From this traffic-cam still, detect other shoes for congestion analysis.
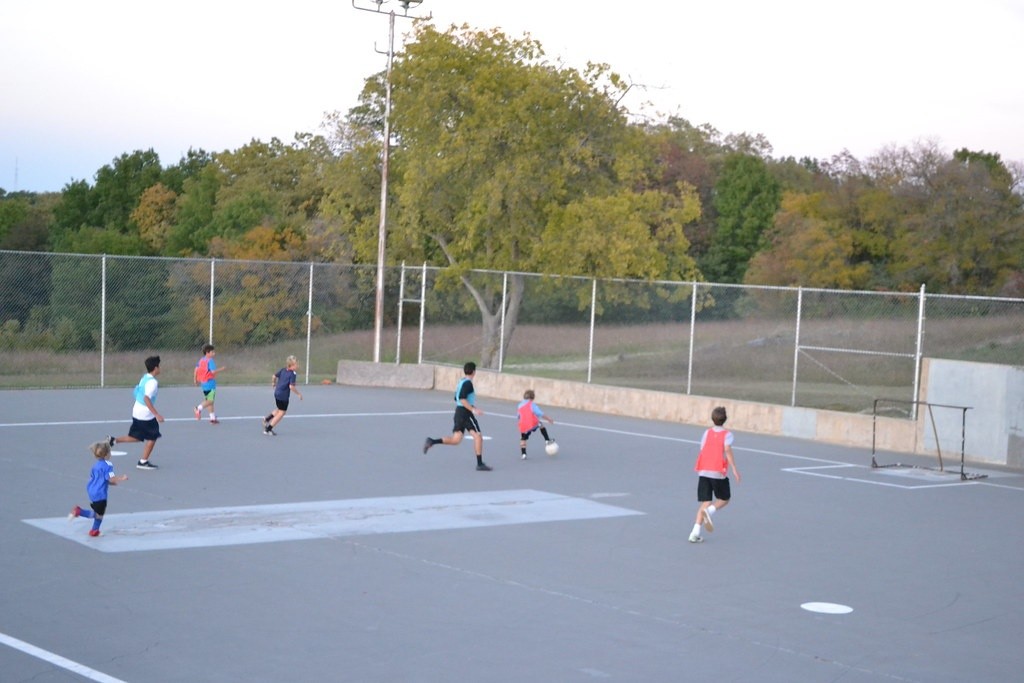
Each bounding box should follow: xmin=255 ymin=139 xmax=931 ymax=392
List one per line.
xmin=546 ymin=438 xmax=555 ymax=445
xmin=68 ymin=506 xmax=80 ymax=521
xmin=521 ymin=454 xmax=526 ymax=459
xmin=263 ymin=430 xmax=277 ymax=436
xmin=263 ymin=416 xmax=268 ymax=428
xmin=89 ymin=530 xmax=104 ymax=537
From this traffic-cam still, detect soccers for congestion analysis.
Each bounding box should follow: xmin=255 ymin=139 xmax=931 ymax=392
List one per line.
xmin=545 ymin=442 xmax=559 ymax=456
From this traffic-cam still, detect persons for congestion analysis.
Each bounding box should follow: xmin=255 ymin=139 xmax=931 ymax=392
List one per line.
xmin=688 ymin=407 xmax=740 ymax=544
xmin=516 ymin=390 xmax=555 ymax=460
xmin=67 ymin=440 xmax=129 ymax=537
xmin=192 ymin=343 xmax=227 ymax=424
xmin=423 ymin=361 xmax=493 ymax=471
xmin=107 ymin=356 xmax=164 ymax=469
xmin=262 ymin=355 xmax=302 ymax=436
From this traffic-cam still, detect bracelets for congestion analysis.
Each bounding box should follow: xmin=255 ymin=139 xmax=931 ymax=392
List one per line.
xmin=472 ymin=408 xmax=476 ymax=413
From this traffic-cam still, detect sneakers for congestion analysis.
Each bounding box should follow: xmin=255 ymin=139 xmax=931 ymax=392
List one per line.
xmin=210 ymin=420 xmax=219 ymax=424
xmin=476 ymin=463 xmax=492 ymax=470
xmin=689 ymin=534 xmax=703 ymax=542
xmin=136 ymin=461 xmax=158 ymax=470
xmin=423 ymin=438 xmax=432 ymax=454
xmin=701 ymin=509 xmax=713 ymax=532
xmin=106 ymin=435 xmax=115 ymax=448
xmin=194 ymin=406 xmax=201 ymax=420
